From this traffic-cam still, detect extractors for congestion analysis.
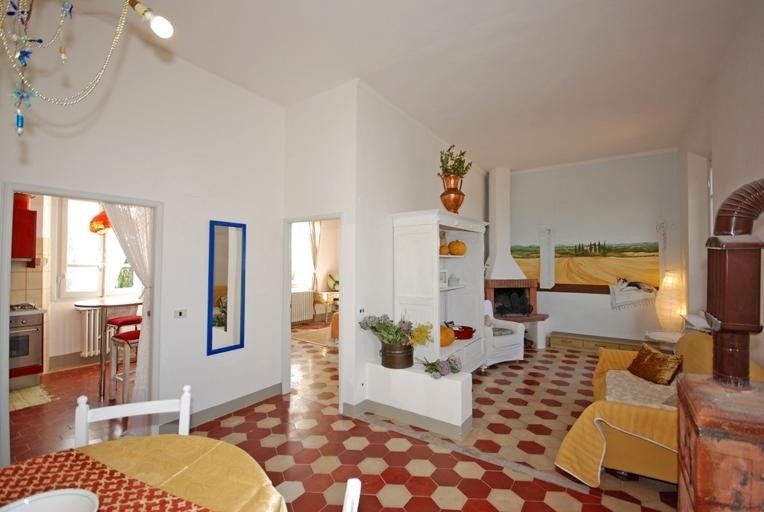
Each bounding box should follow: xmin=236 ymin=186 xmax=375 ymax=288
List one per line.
xmin=11 ymin=193 xmax=32 ymax=262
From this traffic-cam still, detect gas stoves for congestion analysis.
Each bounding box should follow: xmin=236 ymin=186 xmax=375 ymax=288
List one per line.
xmin=8 ymin=303 xmax=42 ymax=328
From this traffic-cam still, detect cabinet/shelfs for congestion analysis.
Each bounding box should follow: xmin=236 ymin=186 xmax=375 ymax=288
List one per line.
xmin=549 ymin=331 xmax=656 ymax=352
xmin=390 ymin=209 xmax=489 ymax=379
xmin=11 ymin=209 xmax=36 ymax=268
xmin=313 ymin=291 xmax=340 ymax=325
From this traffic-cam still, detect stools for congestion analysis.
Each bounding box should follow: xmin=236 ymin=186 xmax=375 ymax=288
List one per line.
xmin=110 ymin=330 xmax=141 ymax=403
xmin=104 ymin=315 xmax=142 ymax=362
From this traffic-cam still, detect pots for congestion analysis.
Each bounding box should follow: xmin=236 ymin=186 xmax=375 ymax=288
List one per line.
xmin=444 ymin=320 xmax=476 ymax=339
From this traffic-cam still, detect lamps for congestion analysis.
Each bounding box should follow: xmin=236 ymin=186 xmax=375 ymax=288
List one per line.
xmin=654 ymin=271 xmax=685 ymax=332
xmin=0 ymin=0 xmax=174 ymax=136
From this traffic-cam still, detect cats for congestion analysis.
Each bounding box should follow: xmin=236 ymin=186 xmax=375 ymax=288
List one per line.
xmin=616 ymin=277 xmax=656 ymax=293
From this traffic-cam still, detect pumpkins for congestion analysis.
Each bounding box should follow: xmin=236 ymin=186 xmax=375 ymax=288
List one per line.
xmin=440 ymin=325 xmax=454 ymax=346
xmin=448 ymin=240 xmax=466 ymax=255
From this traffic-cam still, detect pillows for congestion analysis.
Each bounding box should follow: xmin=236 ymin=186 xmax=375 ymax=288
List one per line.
xmin=627 ymin=342 xmax=682 ymax=385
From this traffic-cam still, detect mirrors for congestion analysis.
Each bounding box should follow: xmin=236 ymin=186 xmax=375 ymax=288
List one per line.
xmin=205 ymin=218 xmax=248 ymax=356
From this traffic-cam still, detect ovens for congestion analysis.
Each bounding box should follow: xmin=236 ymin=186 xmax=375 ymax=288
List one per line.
xmin=8 ymin=326 xmax=42 ymax=390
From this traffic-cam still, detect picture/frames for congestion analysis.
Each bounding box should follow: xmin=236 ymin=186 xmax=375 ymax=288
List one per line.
xmin=440 ymin=269 xmax=448 ymax=289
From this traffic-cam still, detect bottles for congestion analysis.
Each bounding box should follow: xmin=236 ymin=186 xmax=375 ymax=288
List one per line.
xmin=447 ymin=275 xmax=457 ymax=286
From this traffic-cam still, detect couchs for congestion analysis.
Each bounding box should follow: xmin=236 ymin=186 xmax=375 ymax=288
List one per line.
xmin=553 ymin=331 xmax=763 ymax=489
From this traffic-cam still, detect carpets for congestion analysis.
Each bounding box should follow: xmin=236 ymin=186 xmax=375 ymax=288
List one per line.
xmin=9 ymin=384 xmax=62 ymax=413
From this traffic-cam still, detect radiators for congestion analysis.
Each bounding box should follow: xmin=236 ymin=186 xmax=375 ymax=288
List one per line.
xmin=75 ymin=306 xmax=122 ymax=358
xmin=291 ymin=290 xmax=314 ymax=325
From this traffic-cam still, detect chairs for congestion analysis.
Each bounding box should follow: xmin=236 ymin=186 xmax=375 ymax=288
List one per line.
xmin=71 ymin=384 xmax=190 ymax=448
xmin=324 ymin=312 xmax=339 ymax=358
xmin=483 ymin=300 xmax=525 ymax=366
xmin=339 ymin=478 xmax=365 ymax=511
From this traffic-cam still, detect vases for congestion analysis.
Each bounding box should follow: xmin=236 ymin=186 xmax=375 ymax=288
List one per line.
xmin=436 ymin=172 xmax=465 ymax=214
xmin=377 ymin=341 xmax=415 ymax=370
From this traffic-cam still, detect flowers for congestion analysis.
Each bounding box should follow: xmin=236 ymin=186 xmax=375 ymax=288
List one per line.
xmin=416 ymin=352 xmax=464 ymax=379
xmin=437 ymin=144 xmax=473 ymax=176
xmin=216 ymin=295 xmax=227 ymax=312
xmin=211 ymin=313 xmax=225 ymax=327
xmin=358 ymin=309 xmax=435 ymax=349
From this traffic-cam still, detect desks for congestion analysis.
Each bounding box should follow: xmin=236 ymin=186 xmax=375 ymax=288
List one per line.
xmin=76 ymin=296 xmax=143 ymax=397
